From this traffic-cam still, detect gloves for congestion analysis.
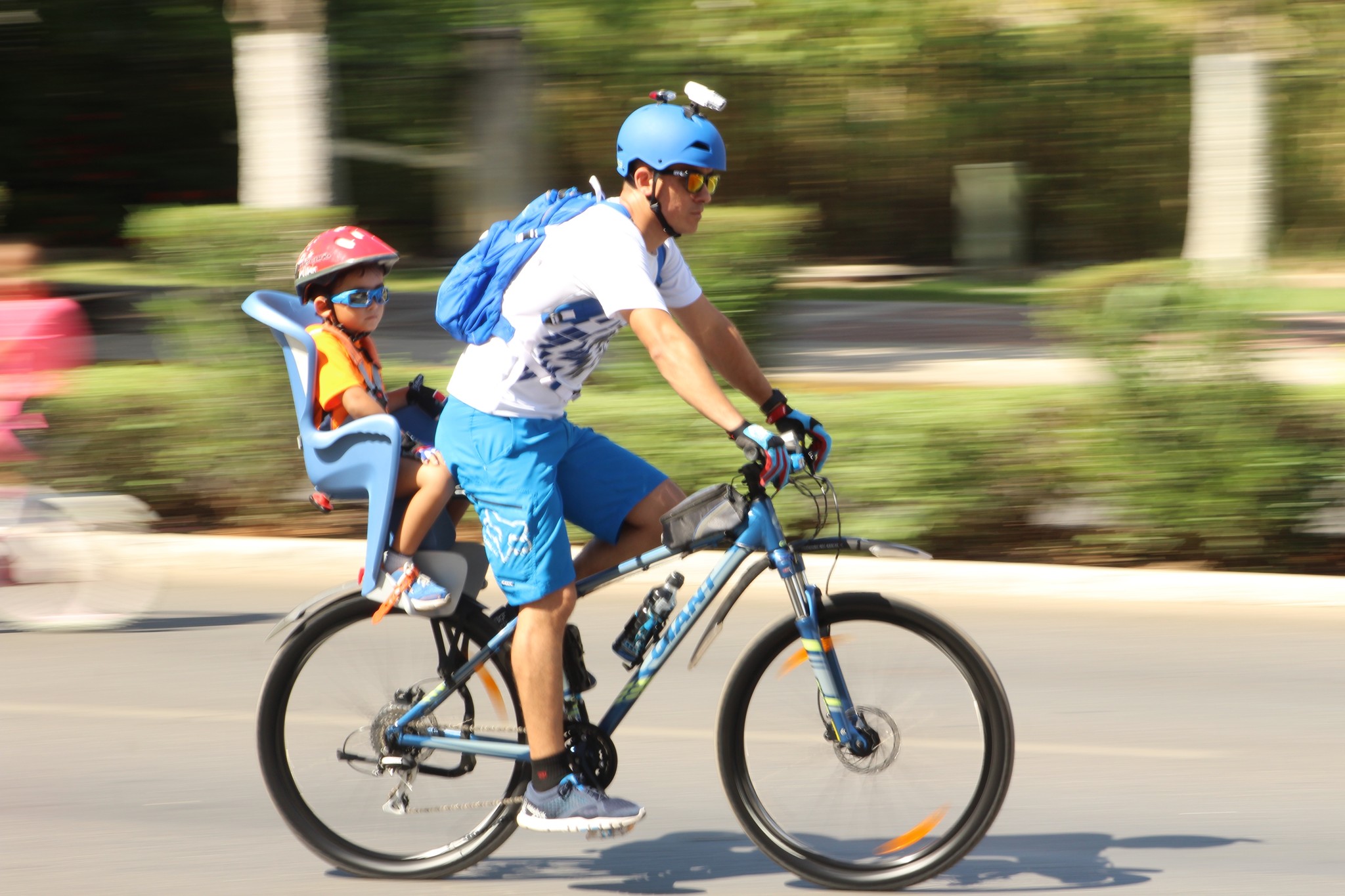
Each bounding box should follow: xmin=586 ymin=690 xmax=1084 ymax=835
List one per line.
xmin=768 ymin=404 xmax=833 ymax=475
xmin=727 ymin=420 xmax=792 ymax=498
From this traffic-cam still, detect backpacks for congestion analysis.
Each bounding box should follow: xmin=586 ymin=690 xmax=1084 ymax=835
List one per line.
xmin=435 ymin=175 xmax=666 ymax=404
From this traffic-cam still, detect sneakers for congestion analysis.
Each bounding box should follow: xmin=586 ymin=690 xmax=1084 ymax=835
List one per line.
xmin=515 ymin=769 xmax=646 ymax=832
xmin=380 ymin=561 xmax=452 ymax=611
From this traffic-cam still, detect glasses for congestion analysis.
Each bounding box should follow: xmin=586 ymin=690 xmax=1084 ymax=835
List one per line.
xmin=649 ymin=167 xmax=721 ymax=195
xmin=330 ymin=287 xmax=390 ymax=308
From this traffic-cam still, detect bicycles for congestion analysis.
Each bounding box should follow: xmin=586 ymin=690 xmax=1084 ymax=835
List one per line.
xmin=257 ymin=426 xmax=1017 ymax=893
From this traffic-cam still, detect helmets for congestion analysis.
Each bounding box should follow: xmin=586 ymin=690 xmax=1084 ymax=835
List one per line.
xmin=293 ymin=225 xmax=403 ymax=306
xmin=615 ymin=102 xmax=727 ymax=178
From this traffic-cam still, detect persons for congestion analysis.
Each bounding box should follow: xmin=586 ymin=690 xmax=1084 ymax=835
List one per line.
xmin=0 ymin=242 xmax=97 ymax=462
xmin=436 ymin=103 xmax=832 ymax=834
xmin=294 ymin=226 xmax=470 ymax=613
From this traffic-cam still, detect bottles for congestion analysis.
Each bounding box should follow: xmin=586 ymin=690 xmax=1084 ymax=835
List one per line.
xmin=610 ymin=571 xmax=685 ymax=669
